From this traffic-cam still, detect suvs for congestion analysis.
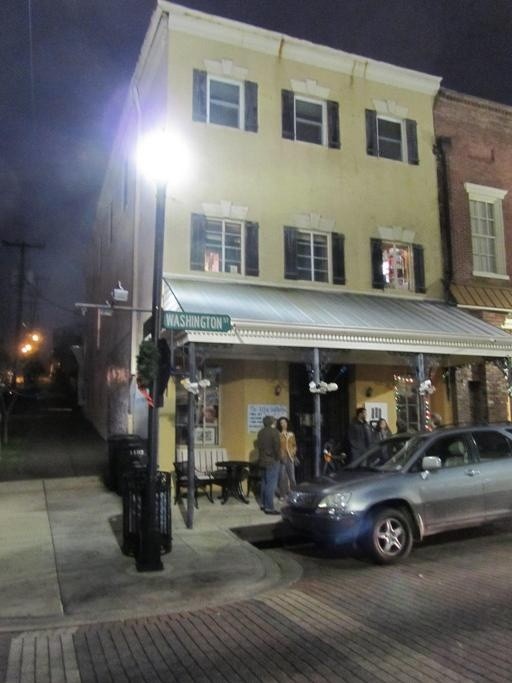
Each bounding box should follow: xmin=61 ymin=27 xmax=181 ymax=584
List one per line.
xmin=280 ymin=421 xmax=511 ymax=563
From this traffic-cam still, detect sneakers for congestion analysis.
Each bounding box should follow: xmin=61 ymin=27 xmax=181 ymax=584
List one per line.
xmin=261 ymin=507 xmax=280 ymax=515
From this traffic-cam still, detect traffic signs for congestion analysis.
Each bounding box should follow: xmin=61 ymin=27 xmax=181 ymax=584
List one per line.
xmin=162 ymin=312 xmax=232 ymax=332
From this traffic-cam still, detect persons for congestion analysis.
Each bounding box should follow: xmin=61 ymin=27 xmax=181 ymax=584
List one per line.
xmin=372 ymin=419 xmax=393 ymax=465
xmin=347 ymin=408 xmax=373 ymax=467
xmin=275 ymin=417 xmax=298 ymax=502
xmin=396 ymin=418 xmax=412 ymax=452
xmin=257 ymin=416 xmax=285 ymax=514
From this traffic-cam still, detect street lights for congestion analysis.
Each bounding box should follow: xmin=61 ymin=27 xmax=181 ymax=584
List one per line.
xmin=129 ymin=119 xmax=200 ymax=574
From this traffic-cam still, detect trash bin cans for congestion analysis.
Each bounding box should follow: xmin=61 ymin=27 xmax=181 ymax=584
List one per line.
xmin=107 ymin=434 xmax=172 ymax=558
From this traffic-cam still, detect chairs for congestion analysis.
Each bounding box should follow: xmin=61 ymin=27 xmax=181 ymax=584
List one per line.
xmin=173 ymin=459 xmax=261 ymax=510
